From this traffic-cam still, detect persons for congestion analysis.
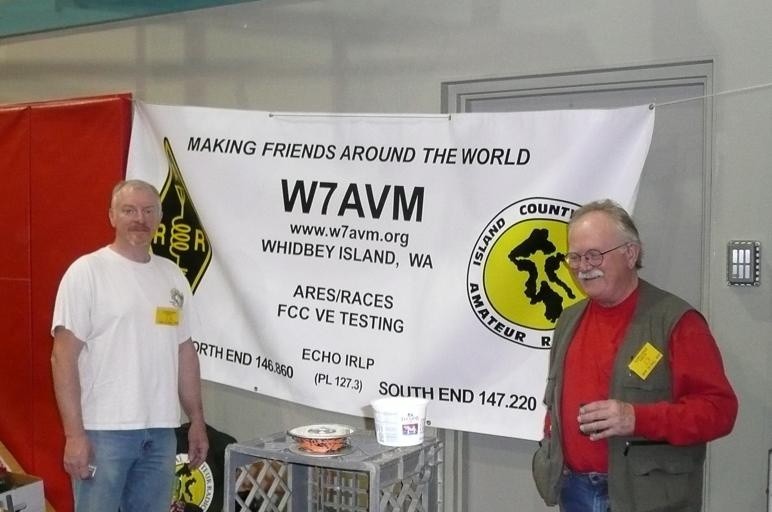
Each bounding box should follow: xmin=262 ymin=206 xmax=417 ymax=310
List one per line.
xmin=528 ymin=197 xmax=740 ymax=512
xmin=47 ymin=177 xmax=210 ymax=509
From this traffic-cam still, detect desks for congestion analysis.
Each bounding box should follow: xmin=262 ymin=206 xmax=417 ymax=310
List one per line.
xmin=220 ymin=422 xmax=444 ymax=511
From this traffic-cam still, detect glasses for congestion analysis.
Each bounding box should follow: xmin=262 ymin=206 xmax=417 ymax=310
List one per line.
xmin=563 ymin=243 xmax=630 ymax=269
